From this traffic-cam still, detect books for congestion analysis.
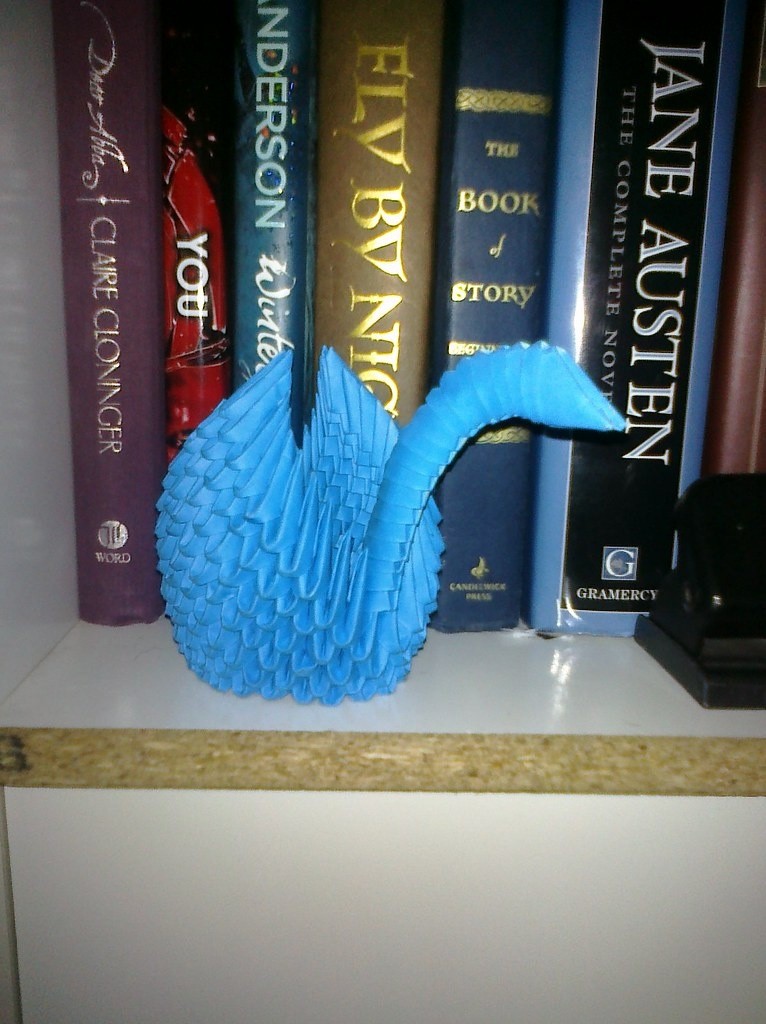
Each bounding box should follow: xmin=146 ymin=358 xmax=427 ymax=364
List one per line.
xmin=50 ymin=0 xmax=766 ymax=638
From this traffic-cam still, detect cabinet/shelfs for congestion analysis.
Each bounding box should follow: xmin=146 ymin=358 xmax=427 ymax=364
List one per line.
xmin=0 ymin=0 xmax=766 ymax=1023
